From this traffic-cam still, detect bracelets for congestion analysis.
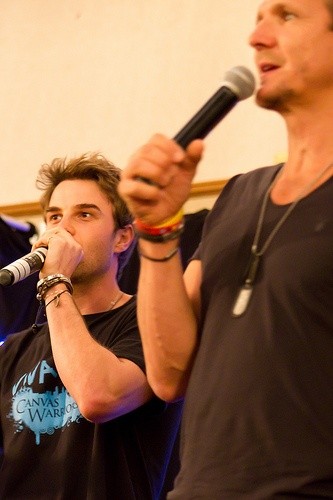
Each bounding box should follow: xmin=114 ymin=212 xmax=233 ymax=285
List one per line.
xmin=32 ymin=273 xmax=73 ymax=327
xmin=134 ymin=245 xmax=186 ymax=265
xmin=130 ymin=218 xmax=188 ymax=244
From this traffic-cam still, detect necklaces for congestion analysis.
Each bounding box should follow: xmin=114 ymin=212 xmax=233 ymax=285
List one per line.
xmin=230 ymin=162 xmax=333 ymax=316
xmin=99 ymin=291 xmax=124 ymax=317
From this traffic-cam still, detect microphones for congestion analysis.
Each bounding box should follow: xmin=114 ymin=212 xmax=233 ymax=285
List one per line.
xmin=0 ymin=246 xmax=47 ymax=287
xmin=133 ymin=66 xmax=256 ymax=188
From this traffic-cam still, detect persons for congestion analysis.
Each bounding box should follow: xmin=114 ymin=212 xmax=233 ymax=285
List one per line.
xmin=1 ymin=153 xmax=185 ymax=500
xmin=118 ymin=0 xmax=333 ymax=500
